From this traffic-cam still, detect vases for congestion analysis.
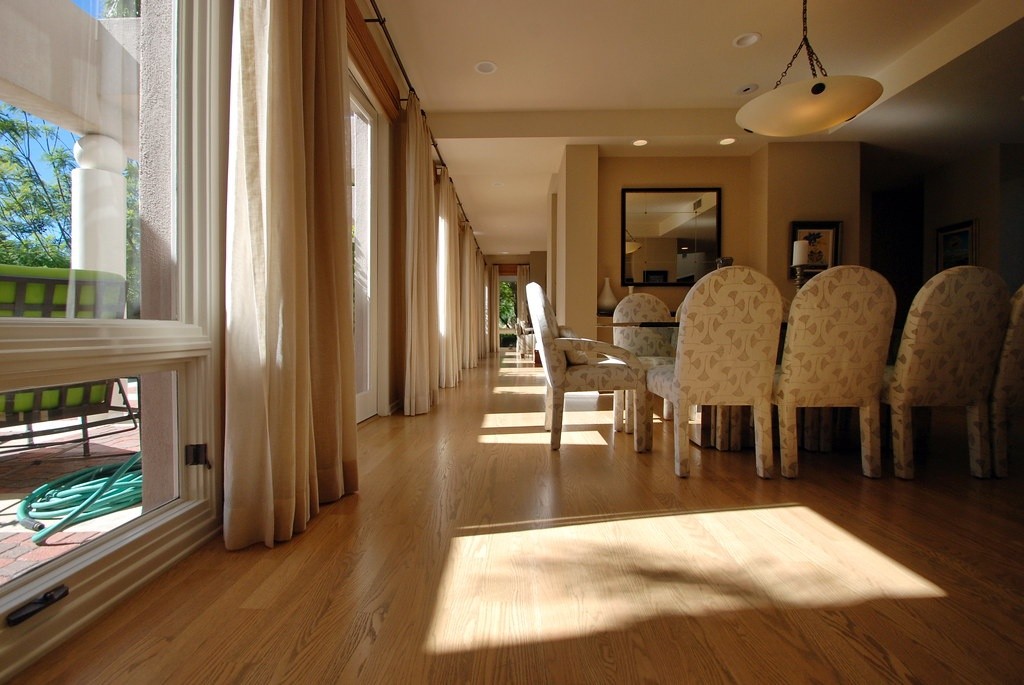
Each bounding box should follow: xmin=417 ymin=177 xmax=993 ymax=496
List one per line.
xmin=598 ymin=277 xmax=619 ymax=314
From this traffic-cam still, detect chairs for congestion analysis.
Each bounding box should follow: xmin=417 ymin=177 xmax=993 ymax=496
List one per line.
xmin=524 ymin=281 xmax=650 ymax=453
xmin=646 ymin=266 xmax=783 ymax=480
xmin=613 ymin=293 xmax=678 ymax=433
xmin=993 ymin=285 xmax=1024 ymax=478
xmin=771 ymin=263 xmax=896 ymax=480
xmin=882 ymin=265 xmax=1012 ymax=483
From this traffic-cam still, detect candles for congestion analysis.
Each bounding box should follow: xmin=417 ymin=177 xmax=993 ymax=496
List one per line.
xmin=790 ymin=239 xmax=810 ymax=293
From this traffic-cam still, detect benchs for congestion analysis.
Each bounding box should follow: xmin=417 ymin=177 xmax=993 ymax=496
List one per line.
xmin=0 ymin=264 xmax=141 ymax=463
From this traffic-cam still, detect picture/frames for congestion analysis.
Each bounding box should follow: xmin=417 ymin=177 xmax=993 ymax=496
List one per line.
xmin=787 ymin=219 xmax=844 ymax=281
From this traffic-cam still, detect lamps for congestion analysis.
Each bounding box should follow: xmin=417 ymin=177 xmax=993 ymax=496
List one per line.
xmin=734 ymin=1 xmax=884 ymax=138
xmin=625 ymin=229 xmax=641 ymax=255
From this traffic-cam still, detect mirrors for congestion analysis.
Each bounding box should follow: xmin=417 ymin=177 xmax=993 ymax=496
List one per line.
xmin=621 ymin=186 xmax=724 ymax=288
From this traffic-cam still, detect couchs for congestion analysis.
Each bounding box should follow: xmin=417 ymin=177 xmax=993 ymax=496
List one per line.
xmin=515 ymin=317 xmax=536 ymax=359
